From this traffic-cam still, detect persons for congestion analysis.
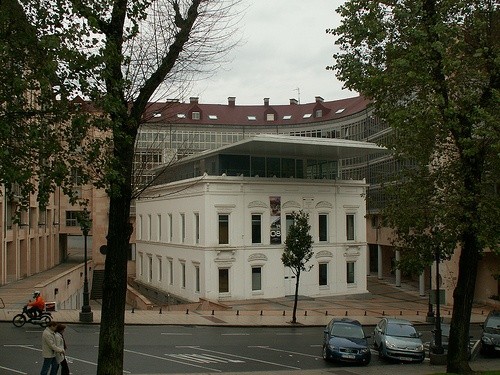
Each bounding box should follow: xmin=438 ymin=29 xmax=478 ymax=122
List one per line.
xmin=54 ymin=325 xmax=70 ymax=375
xmin=27 ymin=291 xmax=45 ymax=318
xmin=40 ymin=321 xmax=64 ymax=375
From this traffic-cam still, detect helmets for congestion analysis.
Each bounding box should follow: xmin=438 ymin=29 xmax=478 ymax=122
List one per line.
xmin=33 ymin=291 xmax=40 ymax=297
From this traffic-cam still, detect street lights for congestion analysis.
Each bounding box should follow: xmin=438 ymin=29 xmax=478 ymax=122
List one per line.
xmin=79 ymin=218 xmax=94 ymax=323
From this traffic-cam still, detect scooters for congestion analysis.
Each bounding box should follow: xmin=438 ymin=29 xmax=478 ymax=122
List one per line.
xmin=12 ymin=300 xmax=54 ymax=328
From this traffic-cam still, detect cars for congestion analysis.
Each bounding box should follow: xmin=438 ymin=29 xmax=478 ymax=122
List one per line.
xmin=323 ymin=317 xmax=371 ymax=367
xmin=479 ymin=309 xmax=500 ymax=361
xmin=373 ymin=318 xmax=426 ymax=366
